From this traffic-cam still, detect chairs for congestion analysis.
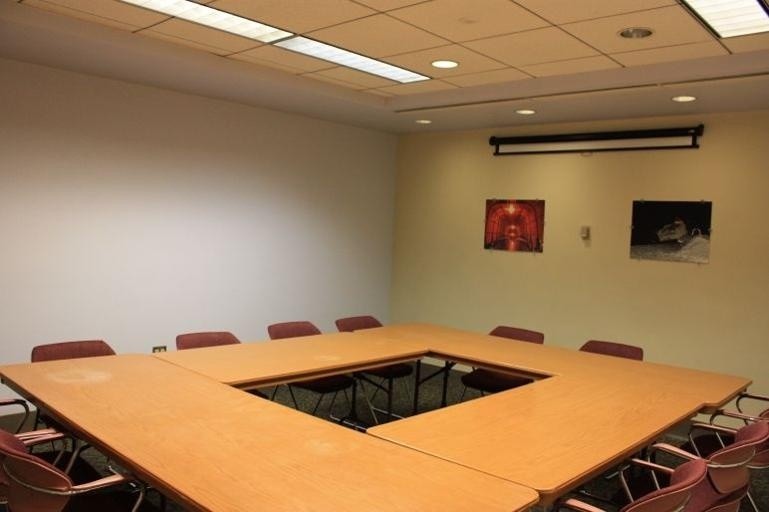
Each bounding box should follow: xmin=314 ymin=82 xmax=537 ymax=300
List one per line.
xmin=333 ymin=315 xmax=416 ymax=420
xmin=30 ymin=340 xmax=117 ymax=465
xmin=268 ymin=321 xmax=378 ymax=431
xmin=681 ymin=390 xmax=768 ymax=470
xmin=579 ymin=339 xmax=645 ymax=360
xmin=1 ymin=399 xmax=91 ymax=465
xmin=554 ymin=455 xmax=708 ymax=512
xmin=490 ymin=325 xmax=544 ymax=345
xmin=178 ymin=332 xmax=269 ymax=399
xmin=639 ymin=421 xmax=768 ymax=511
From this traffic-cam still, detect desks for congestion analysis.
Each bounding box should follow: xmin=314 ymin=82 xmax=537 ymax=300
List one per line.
xmin=366 ymin=375 xmax=706 ymax=510
xmin=150 ymin=331 xmax=429 ymax=390
xmin=353 ymin=321 xmax=754 ymax=415
xmin=1 ymin=352 xmax=541 ymax=511
